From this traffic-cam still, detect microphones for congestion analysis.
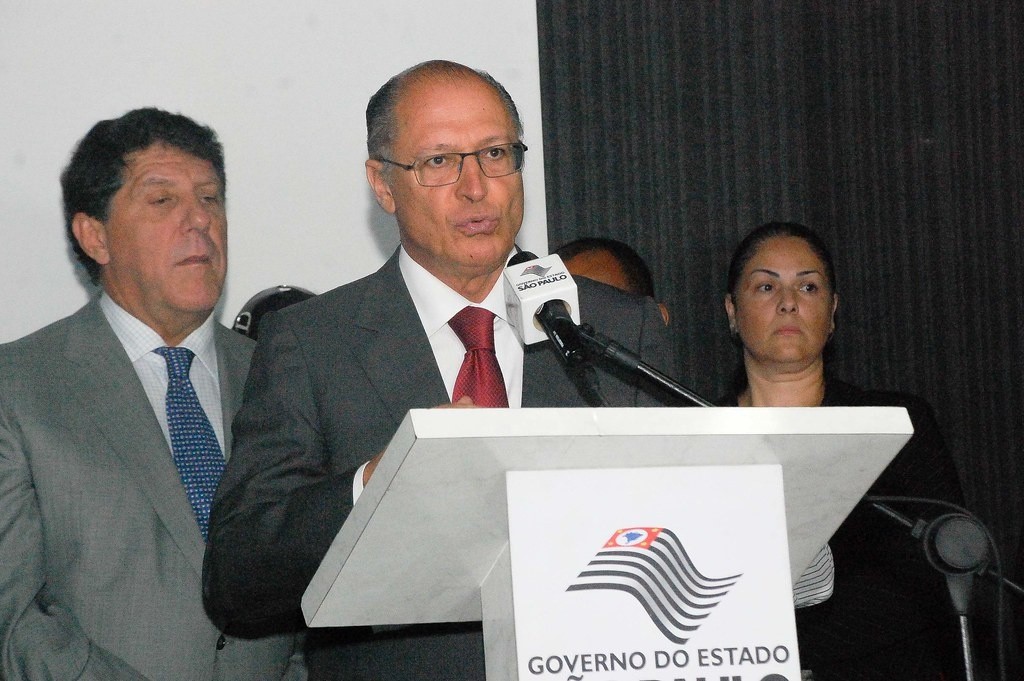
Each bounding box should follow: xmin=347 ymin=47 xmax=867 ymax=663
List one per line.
xmin=500 ymin=251 xmax=587 ymax=368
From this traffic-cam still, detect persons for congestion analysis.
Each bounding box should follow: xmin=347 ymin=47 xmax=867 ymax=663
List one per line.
xmin=0 ymin=108 xmax=310 ymax=681
xmin=710 ymin=220 xmax=997 ymax=681
xmin=200 ymin=60 xmax=681 ymax=681
xmin=551 ymin=236 xmax=669 ymax=327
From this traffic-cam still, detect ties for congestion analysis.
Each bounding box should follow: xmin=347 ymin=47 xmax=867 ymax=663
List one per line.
xmin=447 ymin=307 xmax=509 ymax=409
xmin=151 ymin=347 xmax=228 ymax=542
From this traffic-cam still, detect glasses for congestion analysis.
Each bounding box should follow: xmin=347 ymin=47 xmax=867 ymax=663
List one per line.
xmin=379 ymin=140 xmax=528 ymax=187
